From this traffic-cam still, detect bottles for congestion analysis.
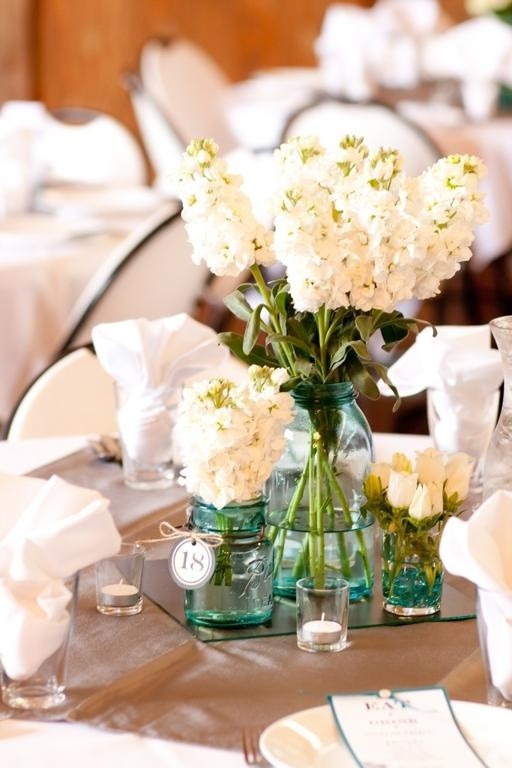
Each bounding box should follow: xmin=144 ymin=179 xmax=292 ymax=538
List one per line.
xmin=484 ymin=315 xmax=512 ymax=500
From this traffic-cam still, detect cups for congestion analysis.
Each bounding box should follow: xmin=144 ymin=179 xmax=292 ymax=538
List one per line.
xmin=114 ymin=378 xmax=179 ymax=489
xmin=296 ymin=577 xmax=349 ymax=654
xmin=1 ymin=568 xmax=78 ymax=709
xmin=94 ymin=543 xmax=146 ymax=616
xmin=427 ymin=389 xmax=500 ymax=493
xmin=475 ymin=585 xmax=512 ymax=708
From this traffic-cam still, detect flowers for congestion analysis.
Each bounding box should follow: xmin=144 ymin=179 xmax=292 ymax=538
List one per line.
xmin=173 ymin=135 xmax=489 ymax=593
xmin=362 ymin=442 xmax=476 ymax=600
xmin=172 ymin=365 xmax=296 ymax=585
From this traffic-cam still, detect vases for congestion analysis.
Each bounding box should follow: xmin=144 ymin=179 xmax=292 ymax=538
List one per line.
xmin=183 ymin=499 xmax=275 ymax=628
xmin=260 ymin=381 xmax=375 ymax=600
xmin=377 ymin=529 xmax=443 ymax=619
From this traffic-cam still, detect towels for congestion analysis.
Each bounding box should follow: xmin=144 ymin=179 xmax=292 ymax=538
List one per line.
xmin=439 ymin=491 xmax=512 ymax=706
xmin=376 ymin=324 xmax=505 ymax=471
xmin=91 ymin=311 xmax=251 ymax=466
xmin=0 ymin=473 xmax=121 ymax=684
